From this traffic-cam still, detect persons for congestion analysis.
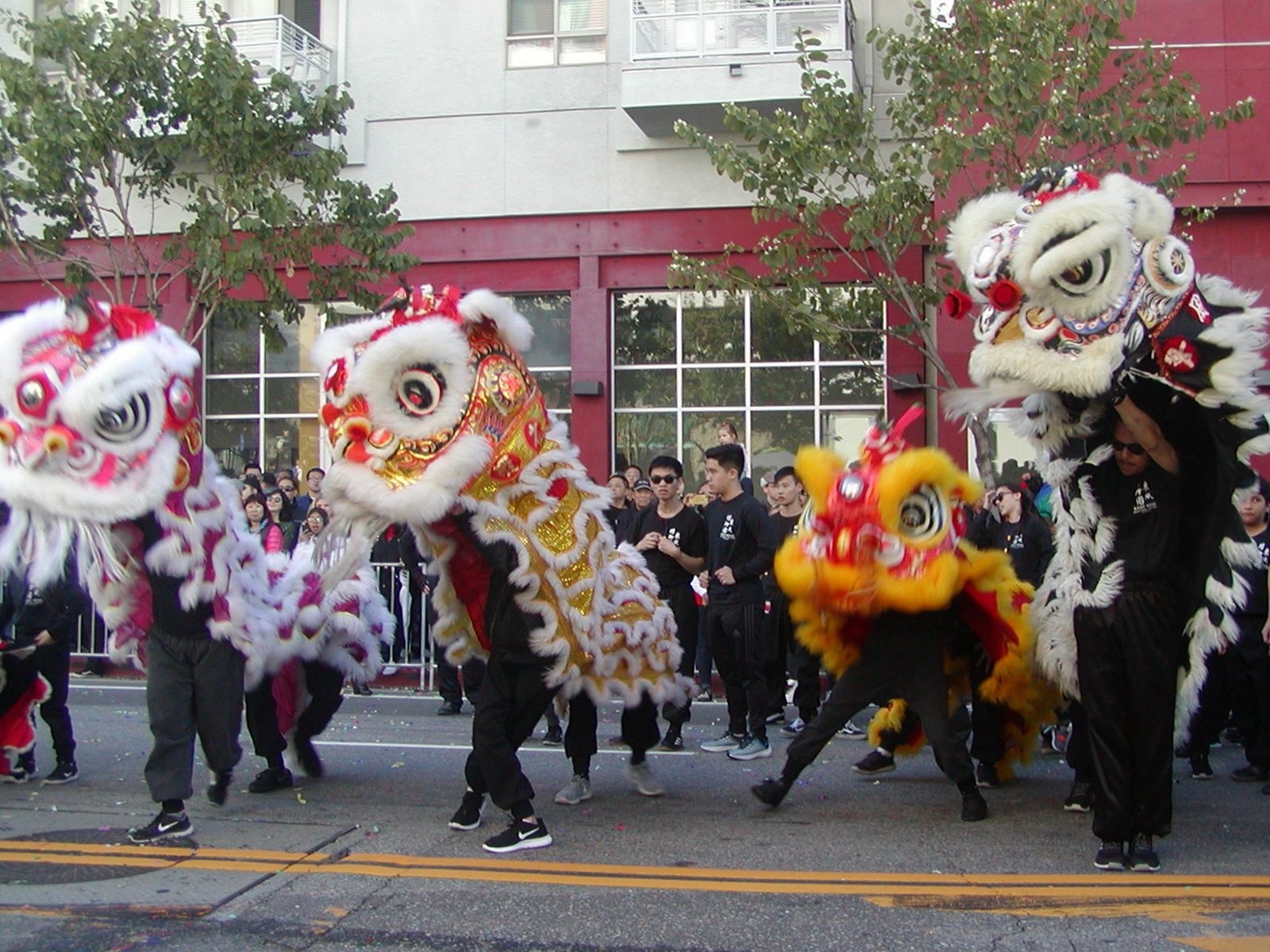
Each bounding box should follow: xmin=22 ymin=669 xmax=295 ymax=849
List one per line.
xmin=602 ymin=464 xmax=652 ymax=548
xmin=245 ymin=658 xmax=345 ymax=794
xmin=700 ymin=445 xmax=775 ymax=760
xmin=555 ymin=689 xmax=664 ymax=804
xmin=760 ymin=468 xmax=869 ymax=739
xmin=237 ymin=464 xmax=328 ymax=559
xmin=678 ymin=474 xmax=753 ymax=703
xmin=0 ymin=502 xmax=110 ymax=784
xmin=1063 ymin=700 xmax=1098 ymax=813
xmin=752 ymin=595 xmax=1010 ymax=821
xmin=1065 ymin=382 xmax=1193 ymax=871
xmin=448 ymin=510 xmax=567 ymax=854
xmin=1177 ymin=476 xmax=1270 ymax=795
xmin=128 ymin=512 xmax=249 ymax=842
xmin=969 ymin=469 xmax=1074 ymax=752
xmin=352 ymin=524 xmax=486 ymax=715
xmin=610 ymin=455 xmax=709 ymax=750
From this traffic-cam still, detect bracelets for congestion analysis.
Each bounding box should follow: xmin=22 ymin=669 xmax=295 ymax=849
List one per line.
xmin=1111 ymin=392 xmax=1126 ymax=405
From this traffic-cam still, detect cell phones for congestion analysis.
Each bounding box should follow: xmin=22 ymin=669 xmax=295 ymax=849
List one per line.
xmin=302 ymin=521 xmax=309 ymax=534
xmin=688 ymin=494 xmax=708 ymax=504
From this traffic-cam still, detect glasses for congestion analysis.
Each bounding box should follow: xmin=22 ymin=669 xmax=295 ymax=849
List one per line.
xmin=651 ymin=474 xmax=678 ymax=485
xmin=306 ymin=518 xmax=325 ymax=523
xmin=993 ymin=492 xmax=1014 ymax=506
xmin=266 ymin=497 xmax=283 ymax=502
xmin=279 ymin=486 xmax=295 ymax=491
xmin=1110 ymin=435 xmax=1143 ymax=455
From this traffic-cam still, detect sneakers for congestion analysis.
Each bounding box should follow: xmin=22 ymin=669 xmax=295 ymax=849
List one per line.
xmin=851 ymin=750 xmax=896 ymax=775
xmin=1 ymin=736 xmax=325 ymax=842
xmin=447 ymin=790 xmax=484 ymax=830
xmin=834 ymin=720 xmax=868 ymax=740
xmin=1063 ymin=776 xmax=1091 ymax=812
xmin=544 ymin=685 xmax=823 ymax=806
xmin=975 ymin=759 xmax=997 ymax=787
xmin=481 ymin=815 xmax=552 ymax=852
xmin=1093 ymin=836 xmax=1125 ymax=869
xmin=1126 ymin=831 xmax=1160 ymax=871
xmin=1189 ymin=749 xmax=1213 ymax=779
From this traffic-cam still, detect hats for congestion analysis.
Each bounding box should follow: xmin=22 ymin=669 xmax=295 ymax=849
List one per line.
xmin=634 ymin=479 xmax=652 ymax=491
xmin=760 ymin=472 xmax=779 ymax=488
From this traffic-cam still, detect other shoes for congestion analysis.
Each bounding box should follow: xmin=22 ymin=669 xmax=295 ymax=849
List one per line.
xmin=1263 ymin=780 xmax=1270 ymax=796
xmin=1053 ymin=725 xmax=1068 ymax=753
xmin=1231 ymin=765 xmax=1264 ymax=781
xmin=1040 ymin=735 xmax=1055 ymax=754
xmin=349 ymin=645 xmax=464 ymax=715
xmin=961 ymin=788 xmax=985 ymax=822
xmin=67 ymin=670 xmax=102 ymax=679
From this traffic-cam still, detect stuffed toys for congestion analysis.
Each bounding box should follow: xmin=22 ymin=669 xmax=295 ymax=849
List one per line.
xmin=775 ymin=402 xmax=1056 ymax=783
xmin=309 ymin=284 xmax=700 ymax=708
xmin=0 ymin=292 xmax=397 ymax=745
xmin=937 ymin=174 xmax=1270 ymax=742
